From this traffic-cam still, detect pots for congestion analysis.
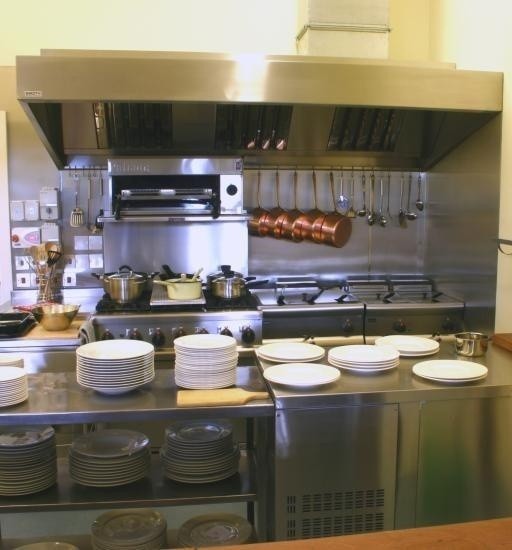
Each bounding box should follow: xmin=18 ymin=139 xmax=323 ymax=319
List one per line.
xmin=92 ymin=265 xmax=269 ymax=303
xmin=249 ymin=169 xmax=352 ymax=248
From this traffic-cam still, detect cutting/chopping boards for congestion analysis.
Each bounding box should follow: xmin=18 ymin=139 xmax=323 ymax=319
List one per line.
xmin=176 ymin=388 xmax=269 ymax=407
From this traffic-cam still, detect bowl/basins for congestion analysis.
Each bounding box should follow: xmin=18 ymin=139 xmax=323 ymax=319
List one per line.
xmin=32 ymin=304 xmax=81 ymax=332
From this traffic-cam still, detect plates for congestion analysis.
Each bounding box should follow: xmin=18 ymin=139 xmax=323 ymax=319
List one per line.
xmin=76 ymin=338 xmax=157 ymax=396
xmin=173 ymin=333 xmax=238 ymax=390
xmin=0 ymin=418 xmax=242 ymax=494
xmin=256 ymin=332 xmax=489 ymax=387
xmin=10 ymin=507 xmax=256 ymax=550
xmin=1 ymin=352 xmax=30 ymax=409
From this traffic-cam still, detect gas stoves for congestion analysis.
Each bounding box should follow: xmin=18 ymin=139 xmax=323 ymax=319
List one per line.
xmin=91 ymin=302 xmax=263 ymax=349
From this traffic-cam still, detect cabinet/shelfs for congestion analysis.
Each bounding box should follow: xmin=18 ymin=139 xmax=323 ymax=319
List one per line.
xmin=1 ymin=365 xmax=275 ymax=550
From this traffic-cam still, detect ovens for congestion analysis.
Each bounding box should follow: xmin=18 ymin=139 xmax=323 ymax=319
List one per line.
xmin=108 ymin=156 xmax=244 ymax=220
